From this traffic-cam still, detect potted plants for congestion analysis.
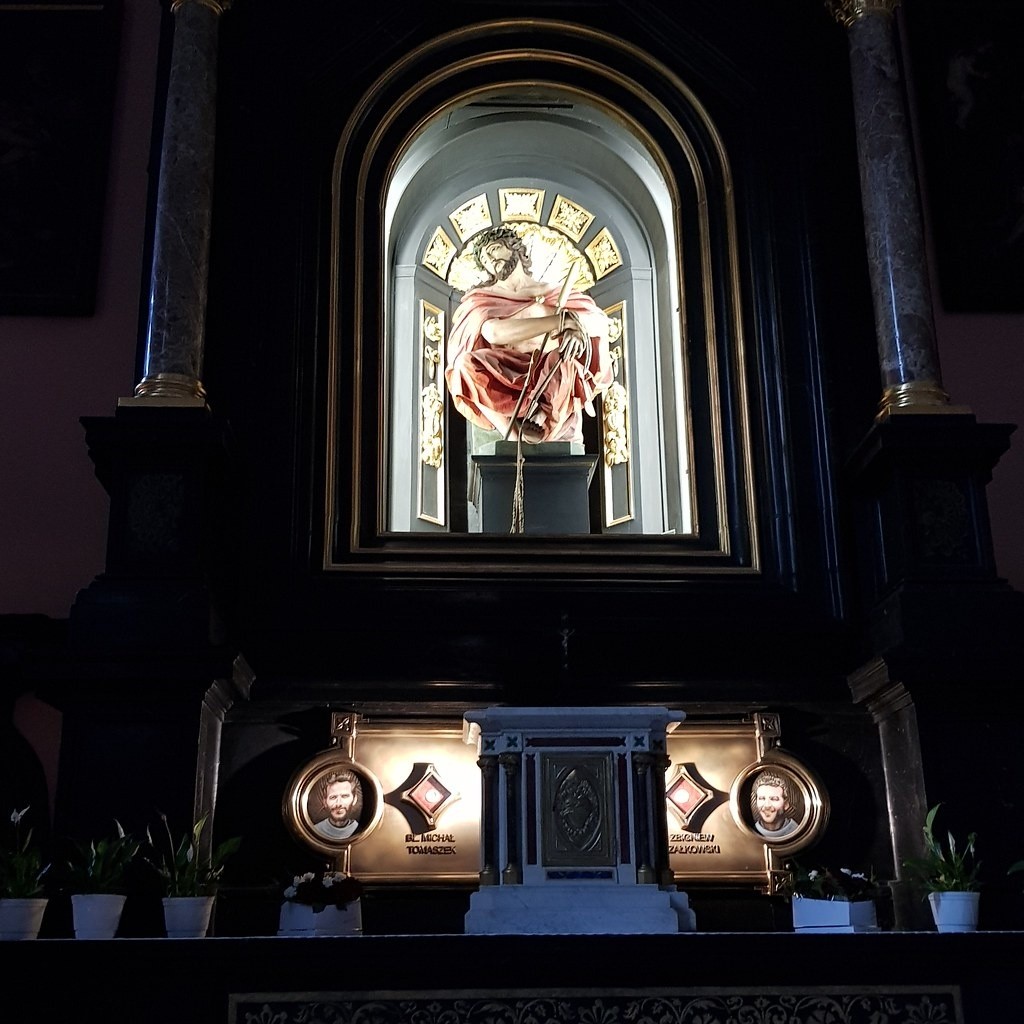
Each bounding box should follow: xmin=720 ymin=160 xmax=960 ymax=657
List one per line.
xmin=141 ymin=809 xmax=243 ymax=937
xmin=904 ymin=801 xmax=989 ymax=932
xmin=60 ymin=818 xmax=139 ymax=938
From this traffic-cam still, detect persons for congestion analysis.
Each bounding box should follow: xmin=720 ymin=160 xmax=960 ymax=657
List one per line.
xmin=437 ymin=229 xmax=613 ymax=443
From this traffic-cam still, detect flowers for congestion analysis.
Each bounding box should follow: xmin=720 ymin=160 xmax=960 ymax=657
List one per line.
xmin=0 ymin=805 xmax=52 ymax=899
xmin=784 ymin=859 xmax=877 ymax=901
xmin=285 ymin=870 xmax=363 ymax=914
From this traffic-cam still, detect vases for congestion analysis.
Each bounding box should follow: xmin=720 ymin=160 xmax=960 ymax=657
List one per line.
xmin=0 ymin=900 xmax=49 ymax=940
